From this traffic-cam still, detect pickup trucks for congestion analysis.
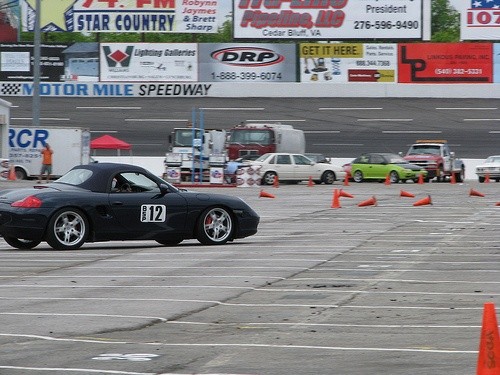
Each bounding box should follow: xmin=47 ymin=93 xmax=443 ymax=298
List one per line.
xmin=398 ymin=143 xmax=465 ymax=182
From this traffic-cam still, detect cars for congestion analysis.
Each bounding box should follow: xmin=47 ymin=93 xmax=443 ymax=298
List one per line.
xmin=343 ymin=151 xmax=427 ymax=183
xmin=254 ymin=153 xmax=346 ymax=185
xmin=475 ymin=155 xmax=500 ymax=183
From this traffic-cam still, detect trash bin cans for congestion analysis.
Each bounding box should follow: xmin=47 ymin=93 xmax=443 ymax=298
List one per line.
xmin=209 ymin=154 xmax=226 ymax=184
xmin=166 ymin=154 xmax=183 ymax=184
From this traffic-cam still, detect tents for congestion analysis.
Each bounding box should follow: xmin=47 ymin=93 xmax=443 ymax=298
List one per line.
xmin=90 ymin=135 xmax=132 ymax=155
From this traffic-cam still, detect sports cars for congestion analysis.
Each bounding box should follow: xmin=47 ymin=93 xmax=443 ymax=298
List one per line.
xmin=0 ymin=162 xmax=259 ymax=250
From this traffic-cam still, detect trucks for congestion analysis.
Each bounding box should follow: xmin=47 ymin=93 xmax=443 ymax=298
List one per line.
xmin=169 ymin=126 xmax=229 ymax=172
xmin=225 ymin=122 xmax=306 ymax=159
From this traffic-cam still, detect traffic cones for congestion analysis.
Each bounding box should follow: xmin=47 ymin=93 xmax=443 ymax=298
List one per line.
xmin=272 ymin=175 xmax=280 ymax=187
xmin=308 ymin=176 xmax=315 ymax=187
xmin=400 ymin=189 xmax=431 ymax=207
xmin=329 ymin=188 xmax=354 ymax=208
xmin=449 ymin=173 xmax=457 ymax=184
xmin=484 ymin=173 xmax=491 ymax=183
xmin=259 ymin=190 xmax=276 ymax=199
xmin=7 ymin=163 xmax=18 ymax=180
xmin=470 ymin=188 xmax=484 ymax=197
xmin=358 ymin=196 xmax=377 ymax=207
xmin=476 ymin=303 xmax=500 ymax=375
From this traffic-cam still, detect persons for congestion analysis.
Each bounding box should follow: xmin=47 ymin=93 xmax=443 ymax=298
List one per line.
xmin=38 ymin=143 xmax=53 ymax=184
